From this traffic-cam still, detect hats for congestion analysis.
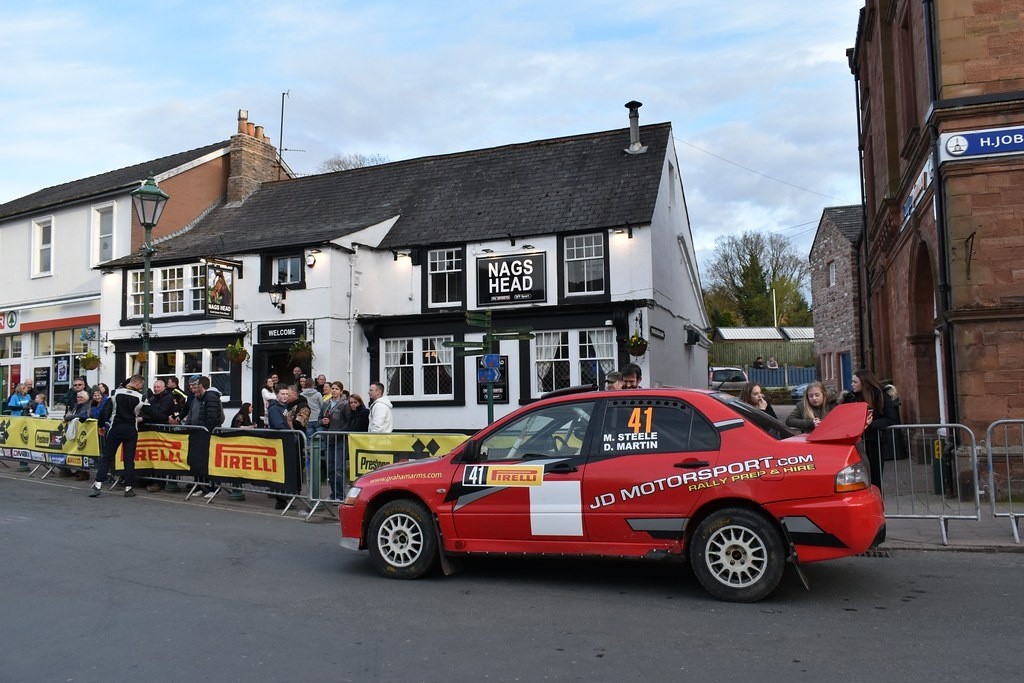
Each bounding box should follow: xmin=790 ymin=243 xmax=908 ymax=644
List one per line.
xmin=91 ymin=384 xmax=105 ymax=397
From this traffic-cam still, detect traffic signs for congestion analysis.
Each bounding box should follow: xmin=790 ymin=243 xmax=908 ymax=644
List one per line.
xmin=466 ymin=318 xmax=487 ymax=327
xmin=495 ymin=325 xmax=535 ymax=334
xmin=480 ymin=354 xmax=501 ymax=369
xmin=493 ymin=333 xmax=536 ymax=342
xmin=441 ymin=340 xmax=485 ymax=348
xmin=477 ymin=367 xmax=502 ymax=384
xmin=464 ymin=310 xmax=486 ymax=320
xmin=454 ymin=349 xmax=486 ymax=357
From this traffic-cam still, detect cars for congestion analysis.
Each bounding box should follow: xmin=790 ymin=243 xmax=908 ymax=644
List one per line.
xmin=336 ymin=384 xmax=891 ymax=606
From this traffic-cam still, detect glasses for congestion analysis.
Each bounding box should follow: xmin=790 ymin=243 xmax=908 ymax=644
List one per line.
xmin=73 ymin=383 xmax=83 ymax=386
xmin=298 ymin=373 xmax=307 ymax=377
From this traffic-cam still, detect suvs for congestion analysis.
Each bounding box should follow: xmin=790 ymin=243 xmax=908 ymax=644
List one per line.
xmin=708 ymin=366 xmax=749 ymax=396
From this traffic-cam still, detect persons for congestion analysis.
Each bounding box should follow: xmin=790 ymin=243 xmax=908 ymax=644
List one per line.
xmin=766 ymin=356 xmax=778 ymax=369
xmin=841 ymin=370 xmax=897 ymax=493
xmin=6 ymin=367 xmax=393 ymax=510
xmin=89 ymin=374 xmax=175 ymax=497
xmin=785 ymin=382 xmax=839 ymax=433
xmin=621 ymin=364 xmax=642 ymax=389
xmin=741 ymin=383 xmax=776 ymax=417
xmin=606 ymin=372 xmax=623 ymax=390
xmin=753 ymin=357 xmax=765 ymax=368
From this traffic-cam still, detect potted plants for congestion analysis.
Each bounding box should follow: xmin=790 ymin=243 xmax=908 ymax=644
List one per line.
xmin=288 ymin=335 xmax=316 ymax=370
xmin=80 ymin=349 xmax=101 ymax=370
xmin=224 ymin=338 xmax=250 ymax=369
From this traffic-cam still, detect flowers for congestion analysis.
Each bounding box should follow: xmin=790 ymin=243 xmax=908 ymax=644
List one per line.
xmin=623 ymin=329 xmax=648 ymax=346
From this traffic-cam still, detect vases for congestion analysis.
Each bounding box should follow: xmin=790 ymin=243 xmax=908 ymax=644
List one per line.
xmin=628 ymin=344 xmax=647 ymax=356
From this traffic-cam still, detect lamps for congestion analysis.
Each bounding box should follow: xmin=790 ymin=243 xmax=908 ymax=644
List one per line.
xmin=306 ymin=248 xmax=322 ymax=268
xmin=268 ymin=281 xmax=285 ymax=313
xmin=704 ymin=327 xmax=712 ymax=333
xmin=615 ymin=227 xmax=624 ymax=233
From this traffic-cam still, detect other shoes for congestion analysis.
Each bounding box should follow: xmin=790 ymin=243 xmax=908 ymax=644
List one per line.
xmin=146 ymin=483 xmax=162 ymax=492
xmin=59 ymin=467 xmax=91 ymax=481
xmin=324 ymin=496 xmax=342 ymax=506
xmin=88 ymin=482 xmax=102 ymax=497
xmin=123 ymin=486 xmax=138 ymax=498
xmin=267 ymin=490 xmax=298 ymax=510
xmin=193 ymin=489 xmax=219 ymax=497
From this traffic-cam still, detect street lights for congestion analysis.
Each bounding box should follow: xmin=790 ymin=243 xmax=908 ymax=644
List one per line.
xmin=129 ymin=170 xmax=171 ymax=405
